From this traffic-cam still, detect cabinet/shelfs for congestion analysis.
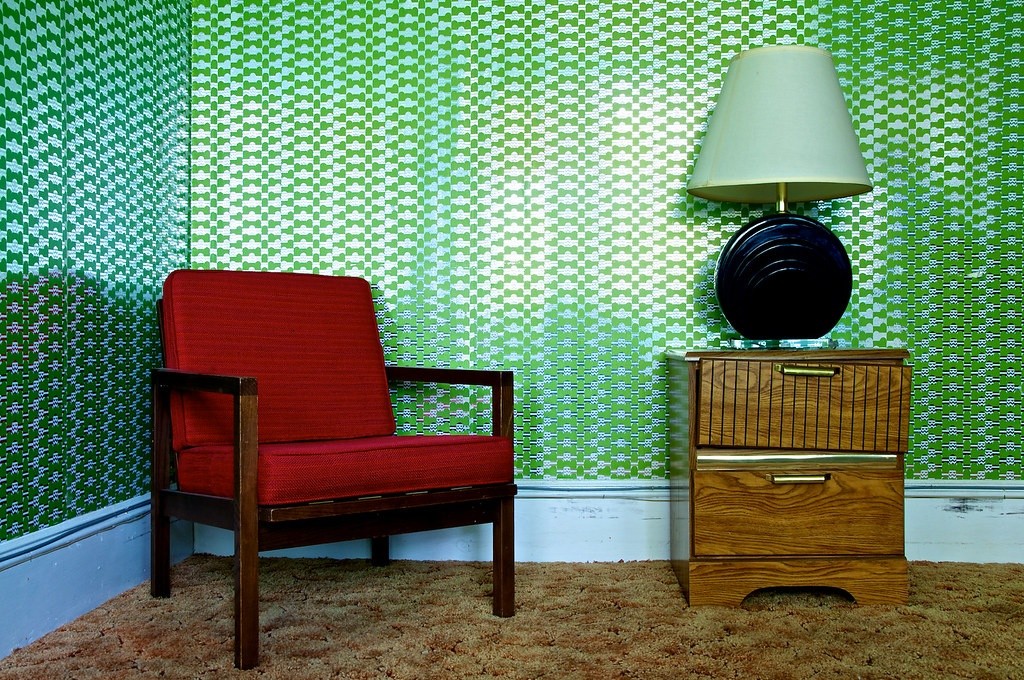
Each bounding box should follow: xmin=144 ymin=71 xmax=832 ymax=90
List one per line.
xmin=662 ymin=346 xmax=912 ymax=608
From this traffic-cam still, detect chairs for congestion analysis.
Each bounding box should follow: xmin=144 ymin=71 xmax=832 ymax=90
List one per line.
xmin=152 ymin=268 xmax=519 ymax=670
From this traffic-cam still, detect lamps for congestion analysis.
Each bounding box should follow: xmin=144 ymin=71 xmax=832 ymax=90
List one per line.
xmin=688 ymin=45 xmax=874 ymax=342
